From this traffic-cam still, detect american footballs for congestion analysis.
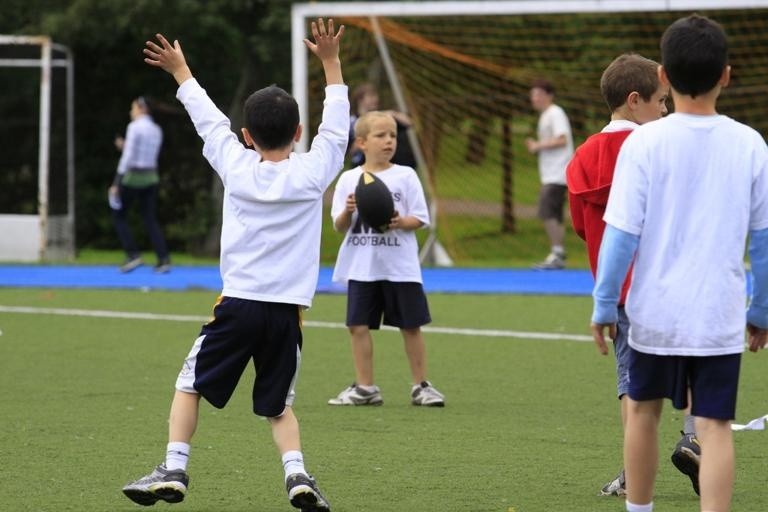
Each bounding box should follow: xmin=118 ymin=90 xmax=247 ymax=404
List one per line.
xmin=356 ymin=174 xmax=395 ymax=233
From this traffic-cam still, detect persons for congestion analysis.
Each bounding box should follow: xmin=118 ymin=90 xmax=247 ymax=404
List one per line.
xmin=594 ymin=11 xmax=767 ymax=512
xmin=109 ymin=95 xmax=175 ymax=275
xmin=117 ymin=18 xmax=351 ymax=511
xmin=326 ymin=110 xmax=446 ymax=410
xmin=342 ymin=82 xmax=418 ymax=171
xmin=566 ymin=51 xmax=707 ymax=502
xmin=526 ymin=82 xmax=589 ymax=272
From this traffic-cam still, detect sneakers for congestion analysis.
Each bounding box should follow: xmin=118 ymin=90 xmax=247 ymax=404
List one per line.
xmin=601 ymin=469 xmax=625 ymax=497
xmin=121 ymin=257 xmax=145 ymax=274
xmin=536 ymin=254 xmax=566 ymax=269
xmin=286 ymin=473 xmax=331 ymax=512
xmin=123 ymin=462 xmax=190 ymax=506
xmin=328 ymin=383 xmax=384 ymax=406
xmin=554 ymin=252 xmax=568 ymax=260
xmin=154 ymin=263 xmax=173 ymax=274
xmin=410 ymin=380 xmax=446 ymax=407
xmin=671 ymin=430 xmax=702 ymax=496
